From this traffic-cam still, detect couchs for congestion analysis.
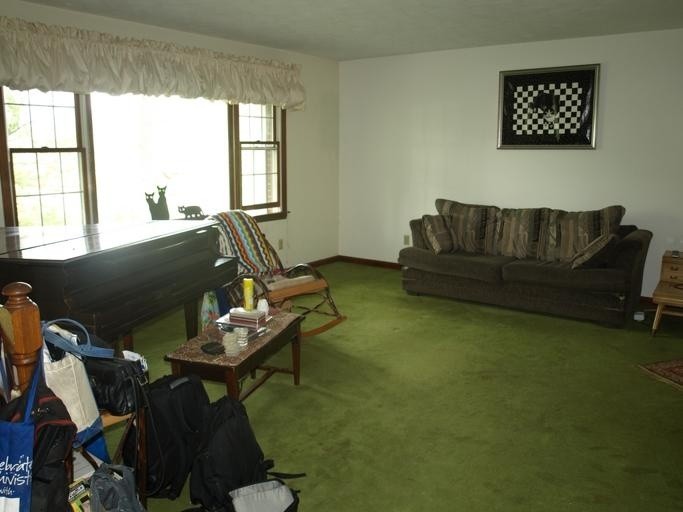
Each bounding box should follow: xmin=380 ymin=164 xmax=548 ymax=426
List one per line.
xmin=399 ymin=219 xmax=652 ymax=330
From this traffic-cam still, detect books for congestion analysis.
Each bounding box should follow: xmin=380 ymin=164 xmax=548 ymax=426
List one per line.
xmin=68 ymin=477 xmax=107 ymax=512
xmin=215 ymin=307 xmax=274 ymax=333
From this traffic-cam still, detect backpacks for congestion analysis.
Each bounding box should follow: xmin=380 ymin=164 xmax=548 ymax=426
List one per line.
xmin=0 ymin=386 xmax=78 ymax=512
xmin=88 ymin=373 xmax=267 ymax=511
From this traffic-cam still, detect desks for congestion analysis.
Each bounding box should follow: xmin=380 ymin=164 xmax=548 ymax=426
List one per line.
xmin=0 ymin=218 xmax=239 ymax=363
xmin=164 ymin=307 xmax=305 ymax=404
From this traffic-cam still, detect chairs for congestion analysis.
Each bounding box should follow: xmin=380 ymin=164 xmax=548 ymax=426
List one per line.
xmin=652 ymin=250 xmax=682 ymax=335
xmin=0 ymin=281 xmax=149 ymax=512
xmin=200 ymin=209 xmax=347 ymax=337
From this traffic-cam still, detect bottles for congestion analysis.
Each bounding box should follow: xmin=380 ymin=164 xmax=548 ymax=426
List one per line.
xmin=257 ymin=298 xmax=269 ymax=319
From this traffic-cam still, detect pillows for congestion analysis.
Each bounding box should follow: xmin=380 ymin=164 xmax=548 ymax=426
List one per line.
xmin=421 ymin=214 xmax=453 ymax=257
xmin=537 ymin=205 xmax=625 ymax=262
xmin=434 ymin=198 xmax=501 ymax=257
xmin=571 ymin=233 xmax=614 ymax=269
xmin=494 ymin=208 xmax=540 ymax=259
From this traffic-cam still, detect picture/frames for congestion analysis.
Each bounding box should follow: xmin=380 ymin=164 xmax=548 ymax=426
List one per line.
xmin=497 ymin=64 xmax=599 ymax=149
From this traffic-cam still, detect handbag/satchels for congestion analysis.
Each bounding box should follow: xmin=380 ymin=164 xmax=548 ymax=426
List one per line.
xmin=83 ymin=355 xmax=151 ymax=414
xmin=0 ymin=421 xmax=36 ymax=512
